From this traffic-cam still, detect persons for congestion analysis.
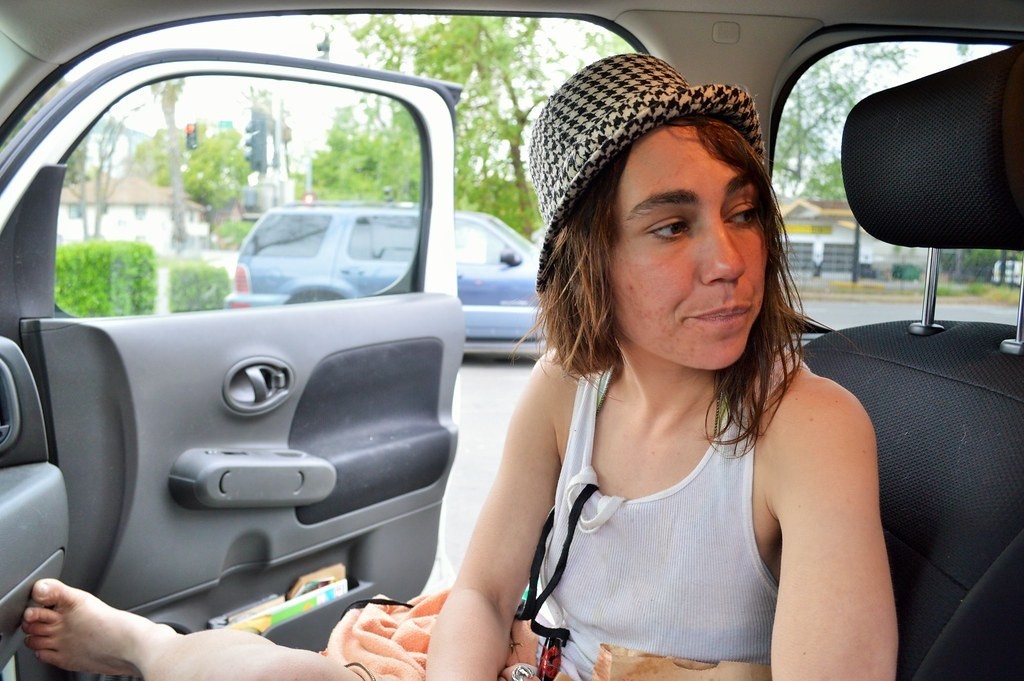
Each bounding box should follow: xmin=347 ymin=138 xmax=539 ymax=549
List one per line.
xmin=23 ymin=54 xmax=895 ymax=681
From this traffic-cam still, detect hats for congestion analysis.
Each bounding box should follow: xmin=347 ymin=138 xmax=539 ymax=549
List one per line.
xmin=529 ymin=53 xmax=766 ymax=296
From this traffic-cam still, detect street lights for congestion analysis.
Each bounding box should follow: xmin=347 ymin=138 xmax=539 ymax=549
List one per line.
xmin=304 ymin=193 xmax=317 ymax=205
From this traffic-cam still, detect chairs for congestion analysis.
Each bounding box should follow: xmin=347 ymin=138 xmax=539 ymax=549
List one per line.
xmin=795 ymin=39 xmax=1024 ymax=681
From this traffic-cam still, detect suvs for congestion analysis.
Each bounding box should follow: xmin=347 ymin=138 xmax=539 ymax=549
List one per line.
xmin=224 ymin=202 xmax=549 ymax=351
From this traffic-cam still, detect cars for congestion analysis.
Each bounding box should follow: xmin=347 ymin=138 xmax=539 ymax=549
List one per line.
xmin=993 ymin=260 xmax=1022 ymax=286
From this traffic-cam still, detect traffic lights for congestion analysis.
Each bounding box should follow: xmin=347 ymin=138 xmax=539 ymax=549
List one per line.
xmin=316 ymin=32 xmax=330 ymax=62
xmin=186 ymin=124 xmax=197 ymax=150
xmin=245 ymin=110 xmax=267 ymax=174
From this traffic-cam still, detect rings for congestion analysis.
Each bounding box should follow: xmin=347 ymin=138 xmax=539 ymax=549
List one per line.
xmin=512 ymin=664 xmax=535 ymax=681
